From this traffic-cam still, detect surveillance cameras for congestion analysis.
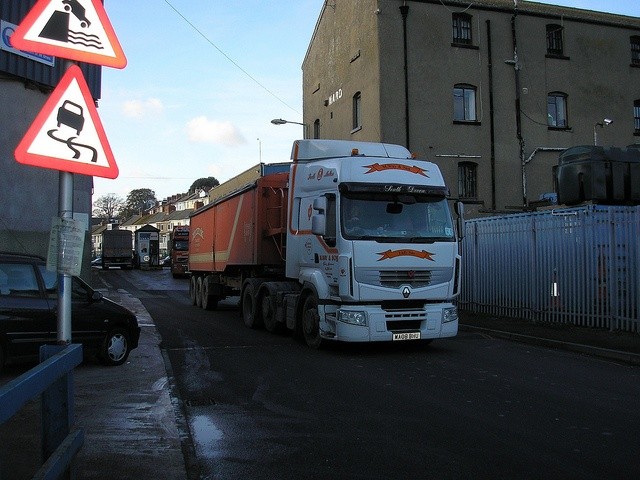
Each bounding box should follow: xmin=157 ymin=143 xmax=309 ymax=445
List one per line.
xmin=603 ymin=117 xmax=614 ymax=125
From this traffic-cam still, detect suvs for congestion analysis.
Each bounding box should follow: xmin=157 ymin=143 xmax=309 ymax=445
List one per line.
xmin=0 ymin=251 xmax=141 ymax=366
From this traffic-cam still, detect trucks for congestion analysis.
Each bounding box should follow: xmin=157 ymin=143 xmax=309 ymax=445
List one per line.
xmin=98 ymin=229 xmax=133 ymax=269
xmin=167 ymin=223 xmax=189 ymax=279
xmin=189 ymin=139 xmax=465 ymax=348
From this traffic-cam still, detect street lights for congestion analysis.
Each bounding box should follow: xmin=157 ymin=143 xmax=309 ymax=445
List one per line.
xmin=271 ymin=118 xmax=308 ymax=139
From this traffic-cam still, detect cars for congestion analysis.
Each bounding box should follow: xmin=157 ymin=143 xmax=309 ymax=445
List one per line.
xmin=91 ymin=258 xmax=102 ymax=265
